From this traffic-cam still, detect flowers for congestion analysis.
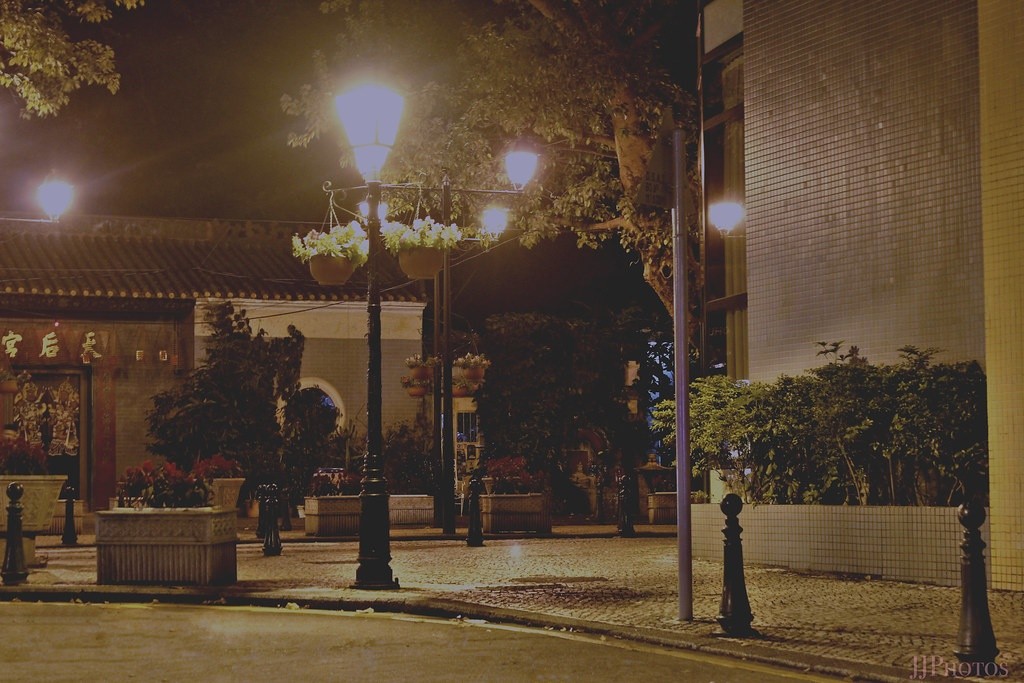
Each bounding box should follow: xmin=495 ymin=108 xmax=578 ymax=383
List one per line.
xmin=483 ymin=455 xmax=538 ymax=492
xmin=310 ymin=467 xmax=371 ymax=494
xmin=379 ymin=216 xmax=463 ymax=257
xmin=291 ymin=219 xmax=370 ymax=270
xmin=452 ymin=376 xmax=478 ymax=392
xmin=403 ymin=351 xmax=440 ymax=368
xmin=452 ymin=351 xmax=491 ymax=368
xmin=113 ymin=453 xmax=241 ymax=507
xmin=400 ymin=374 xmax=430 ymax=388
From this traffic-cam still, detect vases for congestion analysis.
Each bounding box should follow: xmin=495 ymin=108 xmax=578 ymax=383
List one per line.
xmin=409 ymin=366 xmax=433 ymax=378
xmin=303 ymin=496 xmax=361 ymax=535
xmin=480 ymin=493 xmax=545 ymax=533
xmin=309 ymin=253 xmax=355 ymax=286
xmin=94 ymin=507 xmax=239 ymax=584
xmin=451 ymin=366 xmax=484 ymax=380
xmin=407 ymin=385 xmax=426 ymax=396
xmin=451 ymin=384 xmax=480 ymax=397
xmin=397 ymin=245 xmax=445 ymax=278
